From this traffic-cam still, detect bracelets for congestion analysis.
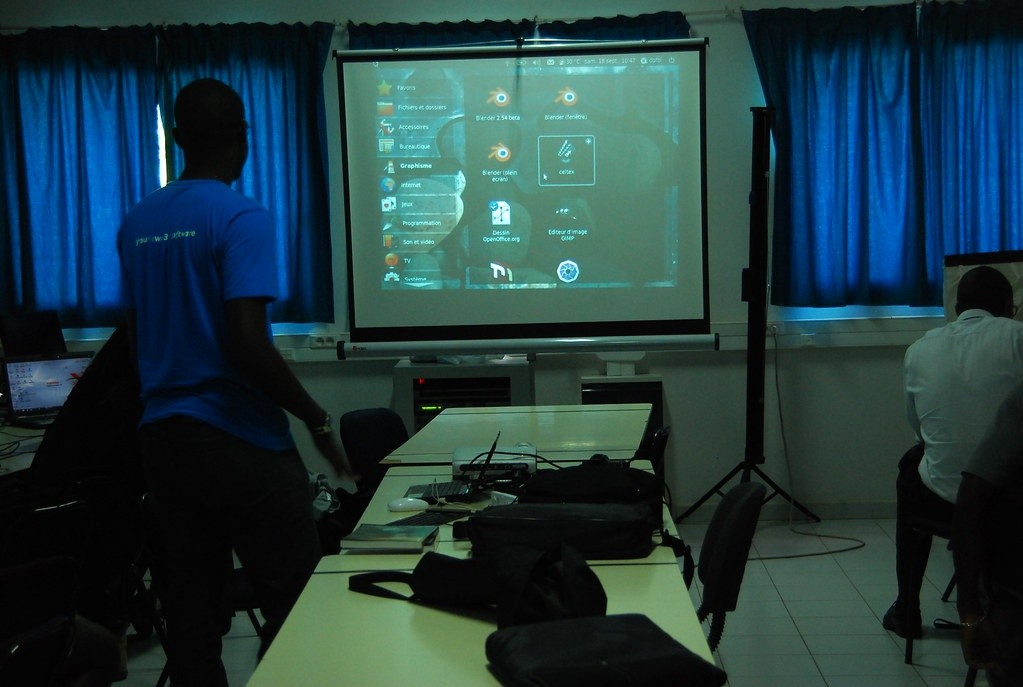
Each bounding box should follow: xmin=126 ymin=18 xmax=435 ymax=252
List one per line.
xmin=963 ymin=615 xmax=985 ymax=630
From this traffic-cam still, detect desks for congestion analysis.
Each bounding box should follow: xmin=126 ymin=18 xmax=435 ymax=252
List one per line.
xmin=339 ymin=459 xmax=680 ymax=555
xmin=244 ymin=545 xmax=715 ymax=687
xmin=379 ymin=403 xmax=651 ymax=464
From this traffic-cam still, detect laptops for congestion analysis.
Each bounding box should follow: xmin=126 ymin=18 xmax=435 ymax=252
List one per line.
xmin=404 ymin=431 xmax=500 ymax=503
xmin=0 ymin=348 xmax=94 ymax=430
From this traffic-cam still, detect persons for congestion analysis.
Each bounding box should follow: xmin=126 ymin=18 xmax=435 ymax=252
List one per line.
xmin=116 ymin=77 xmax=352 ymax=687
xmin=884 ymin=266 xmax=1023 ymax=686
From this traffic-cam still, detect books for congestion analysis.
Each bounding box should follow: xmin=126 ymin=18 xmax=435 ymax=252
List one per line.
xmin=341 ymin=523 xmax=439 ymax=554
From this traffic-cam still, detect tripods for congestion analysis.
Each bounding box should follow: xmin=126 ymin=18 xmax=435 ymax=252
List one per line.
xmin=673 ymin=450 xmax=821 ymax=529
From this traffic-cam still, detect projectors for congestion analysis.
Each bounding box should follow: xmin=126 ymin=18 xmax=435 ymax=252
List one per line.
xmin=452 ymin=441 xmax=538 ymax=481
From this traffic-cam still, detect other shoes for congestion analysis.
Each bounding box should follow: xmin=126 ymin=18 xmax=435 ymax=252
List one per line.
xmin=111 ymin=632 xmax=127 ymax=681
xmin=882 ymin=597 xmax=925 ymax=641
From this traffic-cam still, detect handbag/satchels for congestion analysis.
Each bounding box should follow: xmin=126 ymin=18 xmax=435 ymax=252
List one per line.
xmin=348 ymin=552 xmax=605 ymax=627
xmin=453 ymin=496 xmax=696 ymax=589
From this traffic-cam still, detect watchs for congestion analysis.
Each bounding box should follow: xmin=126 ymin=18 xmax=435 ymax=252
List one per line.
xmin=310 ymin=412 xmax=337 ymax=436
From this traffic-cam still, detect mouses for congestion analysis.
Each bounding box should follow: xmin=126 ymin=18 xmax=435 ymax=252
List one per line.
xmin=388 ymin=497 xmax=428 ymax=511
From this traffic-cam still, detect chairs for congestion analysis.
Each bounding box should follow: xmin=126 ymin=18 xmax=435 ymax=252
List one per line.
xmin=693 ymin=481 xmax=766 ymax=651
xmin=226 ymin=408 xmax=356 ymax=644
xmin=340 ymin=408 xmax=408 ymax=470
xmin=904 ymin=380 xmax=1023 ymax=687
